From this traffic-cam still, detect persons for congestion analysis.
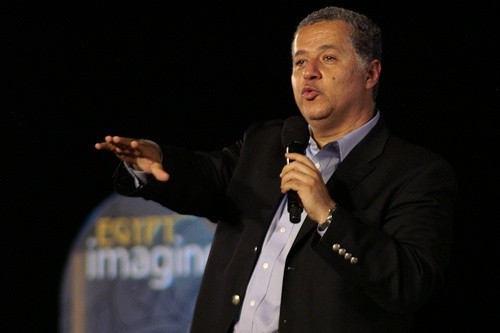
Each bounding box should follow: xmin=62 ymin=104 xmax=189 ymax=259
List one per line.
xmin=91 ymin=6 xmax=457 ymax=333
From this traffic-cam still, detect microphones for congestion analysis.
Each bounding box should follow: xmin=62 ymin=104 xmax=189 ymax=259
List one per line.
xmin=279 ymin=113 xmax=311 ymax=224
